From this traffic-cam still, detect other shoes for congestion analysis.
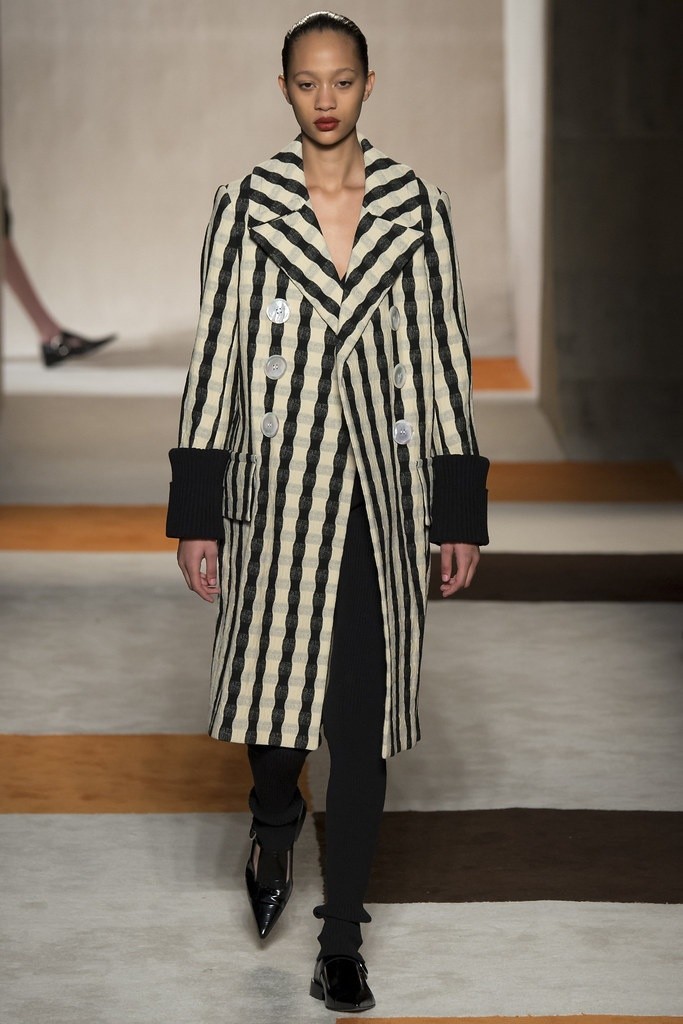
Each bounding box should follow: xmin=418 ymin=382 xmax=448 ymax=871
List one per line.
xmin=41 ymin=330 xmax=118 ymax=366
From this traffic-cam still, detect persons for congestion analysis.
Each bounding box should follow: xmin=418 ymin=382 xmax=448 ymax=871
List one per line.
xmin=165 ymin=10 xmax=490 ymax=1013
xmin=0 ymin=170 xmax=116 ymax=368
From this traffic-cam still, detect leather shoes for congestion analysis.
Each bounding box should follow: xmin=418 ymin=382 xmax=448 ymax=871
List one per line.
xmin=245 ymin=797 xmax=308 ymax=940
xmin=309 ymin=955 xmax=377 ymax=1012
